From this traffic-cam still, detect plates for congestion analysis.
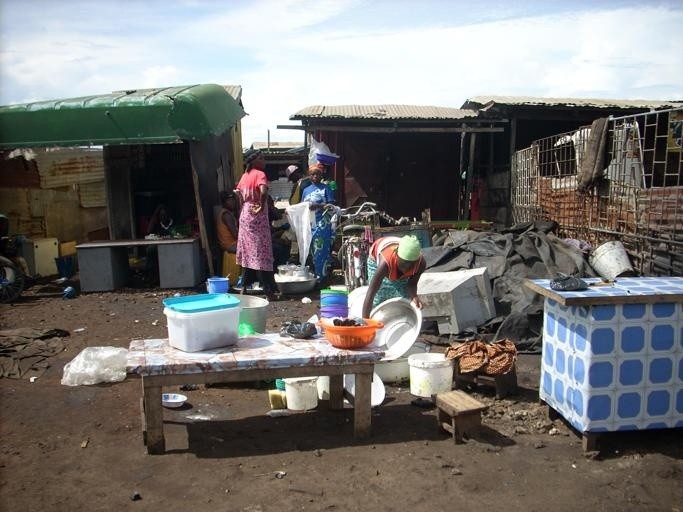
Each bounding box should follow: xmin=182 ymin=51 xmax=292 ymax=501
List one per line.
xmin=453 ymin=331 xmax=475 ymax=342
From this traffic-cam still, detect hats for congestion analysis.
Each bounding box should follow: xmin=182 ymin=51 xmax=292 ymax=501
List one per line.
xmin=285 ymin=165 xmax=299 ymax=183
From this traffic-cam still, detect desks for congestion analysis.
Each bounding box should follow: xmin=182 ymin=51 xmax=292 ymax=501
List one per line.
xmin=126 ymin=334 xmax=385 ymax=455
xmin=74 ymin=238 xmax=201 ymax=292
xmin=525 ymin=276 xmax=683 ymax=452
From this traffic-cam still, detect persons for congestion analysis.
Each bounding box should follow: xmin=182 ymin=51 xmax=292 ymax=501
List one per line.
xmin=144 ymin=210 xmax=181 ymax=286
xmin=145 ymin=203 xmax=170 ymax=237
xmin=214 ymin=149 xmax=334 ymax=295
xmin=361 ymin=233 xmax=424 ymax=320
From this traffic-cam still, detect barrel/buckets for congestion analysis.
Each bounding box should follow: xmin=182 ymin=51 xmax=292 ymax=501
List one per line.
xmin=277 ymin=263 xmax=311 ymax=277
xmin=588 ymin=237 xmax=634 ymax=279
xmin=231 ymin=293 xmax=270 ymax=334
xmin=407 ymin=352 xmax=455 ymax=397
xmin=207 ymin=277 xmax=230 ymax=295
xmin=284 ymin=376 xmax=319 ymax=411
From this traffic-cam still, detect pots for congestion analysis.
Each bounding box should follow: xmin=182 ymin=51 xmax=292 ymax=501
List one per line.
xmin=276 ymin=263 xmax=310 ymax=279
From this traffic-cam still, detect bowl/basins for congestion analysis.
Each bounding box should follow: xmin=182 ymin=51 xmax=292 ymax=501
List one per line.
xmin=343 ymin=371 xmax=384 ymax=410
xmin=274 ymin=274 xmax=320 ymax=295
xmin=158 ymin=392 xmax=186 ymax=410
xmin=231 ymin=285 xmax=263 ymax=296
xmin=316 ymin=152 xmax=337 ymax=163
xmin=319 ymin=288 xmax=350 ymax=319
xmin=366 ymin=296 xmax=421 ymax=361
xmin=373 ymin=342 xmax=432 ymax=382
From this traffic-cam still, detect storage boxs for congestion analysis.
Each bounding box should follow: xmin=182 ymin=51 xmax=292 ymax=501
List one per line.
xmin=163 ymin=294 xmax=242 ymax=353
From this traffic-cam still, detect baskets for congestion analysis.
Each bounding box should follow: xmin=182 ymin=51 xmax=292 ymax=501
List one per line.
xmin=315 ymin=317 xmax=384 ymax=349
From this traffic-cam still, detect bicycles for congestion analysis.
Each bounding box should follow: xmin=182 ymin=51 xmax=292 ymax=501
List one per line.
xmin=0 ymin=238 xmax=30 ymax=305
xmin=319 ymin=200 xmax=379 ymax=293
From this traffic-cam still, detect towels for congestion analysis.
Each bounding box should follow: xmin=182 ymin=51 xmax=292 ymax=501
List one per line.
xmin=397 ymin=235 xmax=420 ymax=261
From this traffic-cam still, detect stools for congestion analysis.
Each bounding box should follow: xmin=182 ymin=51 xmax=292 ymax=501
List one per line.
xmin=431 ymin=389 xmax=489 ymax=445
xmin=453 ymin=360 xmax=517 ymax=400
